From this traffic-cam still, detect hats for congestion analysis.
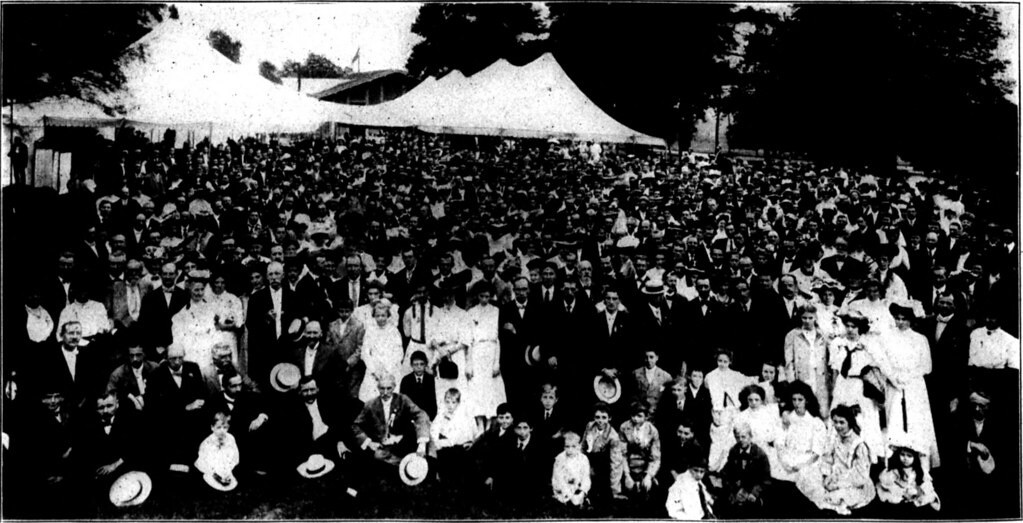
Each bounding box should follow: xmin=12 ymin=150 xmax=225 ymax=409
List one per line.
xmin=889 ymin=440 xmax=926 ymax=458
xmin=109 ymin=471 xmax=152 ymax=507
xmin=296 ymin=454 xmax=335 ymax=477
xmin=889 ymin=299 xmax=925 ymax=321
xmin=398 ymin=452 xmax=429 ymax=487
xmin=288 ymin=316 xmax=308 ymax=342
xmin=270 ymin=363 xmax=301 ymax=392
xmin=524 ymin=344 xmax=540 ymax=366
xmin=641 ymin=278 xmax=669 ymax=293
xmin=836 ymin=308 xmax=867 ymax=319
xmin=203 ymin=467 xmax=237 ymax=491
xmin=594 ymin=374 xmax=621 ymax=404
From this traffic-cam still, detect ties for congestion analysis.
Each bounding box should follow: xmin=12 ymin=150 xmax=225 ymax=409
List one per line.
xmin=743 ymin=305 xmax=748 ymax=312
xmin=417 ymin=378 xmax=422 ymax=389
xmin=544 ymin=289 xmax=549 ymax=304
xmin=840 ymin=345 xmax=857 ymax=379
xmin=518 ymin=443 xmax=523 ymax=453
xmin=545 ymin=412 xmax=549 ymax=422
xmin=566 ymin=305 xmax=571 ymax=318
xmin=130 ymin=288 xmax=137 ymax=313
xmin=698 ymin=483 xmax=711 ymax=519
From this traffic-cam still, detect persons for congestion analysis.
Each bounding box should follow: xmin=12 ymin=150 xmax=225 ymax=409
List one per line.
xmin=1 ymin=128 xmax=1019 ymax=522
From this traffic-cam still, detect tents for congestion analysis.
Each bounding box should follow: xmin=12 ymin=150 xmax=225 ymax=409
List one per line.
xmin=0 ymin=10 xmax=670 ymax=191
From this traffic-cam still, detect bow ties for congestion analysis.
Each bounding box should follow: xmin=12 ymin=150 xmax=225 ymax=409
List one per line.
xmin=701 ymin=299 xmax=708 ymax=306
xmin=584 ymin=285 xmax=590 ymax=290
xmin=666 ymin=295 xmax=672 ymax=301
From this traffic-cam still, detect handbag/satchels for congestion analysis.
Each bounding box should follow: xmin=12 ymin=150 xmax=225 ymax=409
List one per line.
xmin=439 ymin=351 xmax=458 ymax=379
